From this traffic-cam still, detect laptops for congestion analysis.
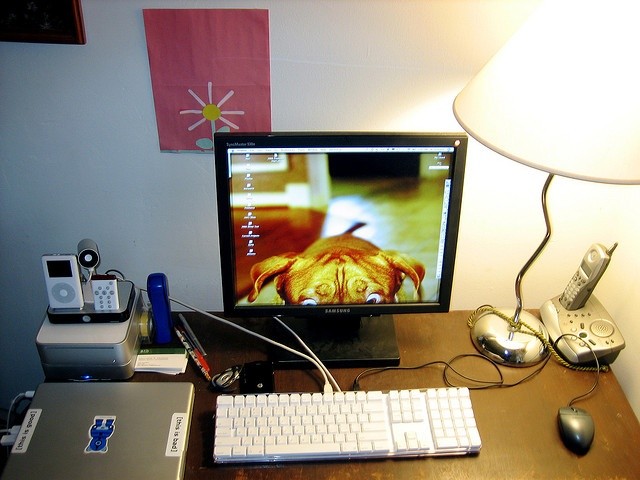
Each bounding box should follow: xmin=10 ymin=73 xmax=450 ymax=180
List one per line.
xmin=2 ymin=382 xmax=196 ymax=480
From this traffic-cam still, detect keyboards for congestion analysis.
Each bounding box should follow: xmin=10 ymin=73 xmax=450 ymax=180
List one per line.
xmin=212 ymin=386 xmax=482 ymax=464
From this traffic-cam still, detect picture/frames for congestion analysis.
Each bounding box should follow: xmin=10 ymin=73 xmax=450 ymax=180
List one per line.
xmin=1 ymin=0 xmax=86 ymax=45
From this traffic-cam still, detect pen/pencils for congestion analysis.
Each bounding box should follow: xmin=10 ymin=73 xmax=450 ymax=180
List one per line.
xmin=173 ymin=326 xmax=211 ymax=382
xmin=178 ymin=326 xmax=210 ymax=374
xmin=178 ymin=312 xmax=208 ymax=357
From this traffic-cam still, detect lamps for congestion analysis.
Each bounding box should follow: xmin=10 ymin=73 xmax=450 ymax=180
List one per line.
xmin=452 ymin=0 xmax=640 ymax=368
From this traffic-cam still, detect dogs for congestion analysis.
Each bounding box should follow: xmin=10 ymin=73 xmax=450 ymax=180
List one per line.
xmin=247 ymin=222 xmax=427 ymax=306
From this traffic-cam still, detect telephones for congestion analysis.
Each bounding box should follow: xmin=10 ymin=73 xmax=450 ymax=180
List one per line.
xmin=539 ymin=242 xmax=627 ymax=363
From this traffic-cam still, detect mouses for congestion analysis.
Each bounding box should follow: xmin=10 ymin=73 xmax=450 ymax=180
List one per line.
xmin=556 ymin=407 xmax=596 ymax=456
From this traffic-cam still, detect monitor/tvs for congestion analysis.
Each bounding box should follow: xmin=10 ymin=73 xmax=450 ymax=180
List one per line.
xmin=212 ymin=130 xmax=469 ymax=370
